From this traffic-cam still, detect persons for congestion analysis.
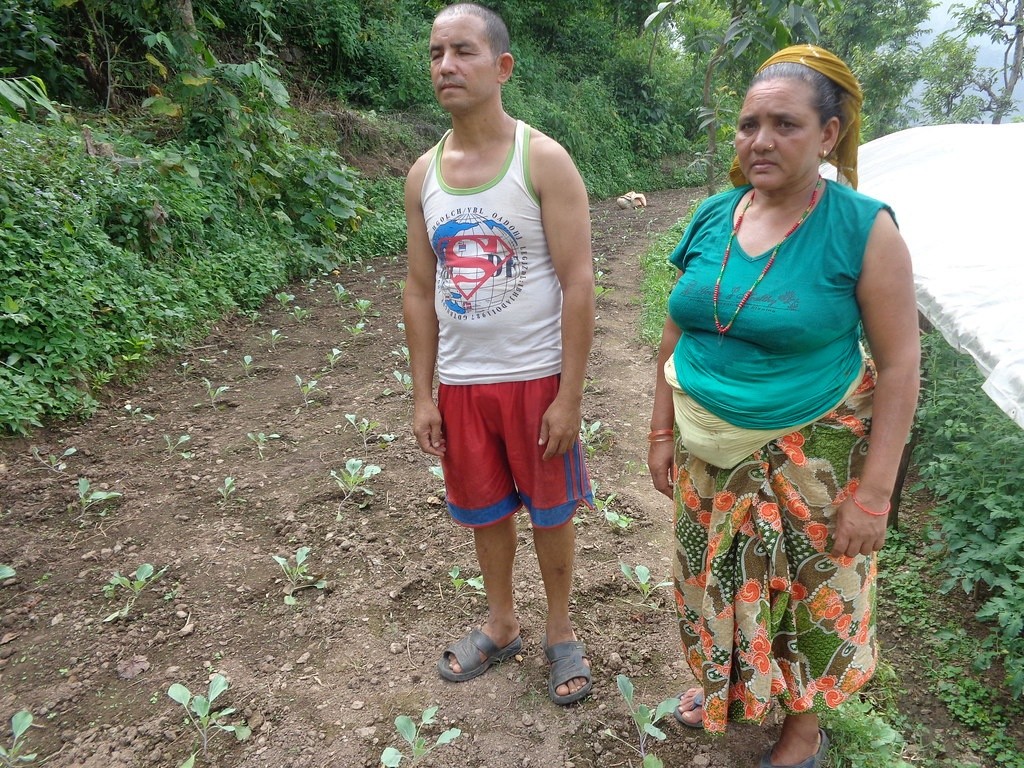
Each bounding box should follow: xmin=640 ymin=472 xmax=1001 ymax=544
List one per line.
xmin=403 ymin=3 xmax=594 ymax=705
xmin=647 ymin=43 xmax=922 ymax=768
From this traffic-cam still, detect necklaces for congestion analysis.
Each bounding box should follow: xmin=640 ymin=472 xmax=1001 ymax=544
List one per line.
xmin=714 ymin=173 xmax=822 ymax=333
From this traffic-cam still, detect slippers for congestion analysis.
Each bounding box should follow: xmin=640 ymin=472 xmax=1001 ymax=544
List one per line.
xmin=544 ymin=634 xmax=591 ymax=701
xmin=674 ymin=688 xmax=709 ymax=726
xmin=437 ymin=624 xmax=523 ymax=681
xmin=759 ymin=729 xmax=832 ymax=768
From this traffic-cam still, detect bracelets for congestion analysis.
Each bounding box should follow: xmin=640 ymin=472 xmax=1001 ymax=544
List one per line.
xmin=852 ymin=493 xmax=891 ymax=516
xmin=647 ymin=430 xmax=675 ymax=442
